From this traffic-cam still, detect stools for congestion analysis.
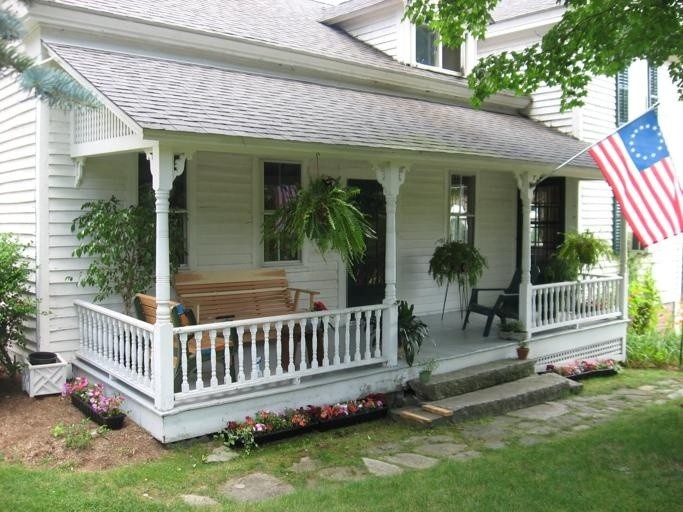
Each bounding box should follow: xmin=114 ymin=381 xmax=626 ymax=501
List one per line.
xmin=278 ymin=322 xmax=325 ymax=373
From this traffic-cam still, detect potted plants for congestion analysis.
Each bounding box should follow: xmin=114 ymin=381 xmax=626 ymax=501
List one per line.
xmin=258 ymin=175 xmax=377 ymax=283
xmin=497 ymin=318 xmax=529 ymax=360
xmin=362 ymin=300 xmax=433 ymax=366
xmin=554 ymin=232 xmax=613 ymax=280
xmin=428 ymin=241 xmax=488 ymax=288
xmin=417 ymin=359 xmax=441 ymax=382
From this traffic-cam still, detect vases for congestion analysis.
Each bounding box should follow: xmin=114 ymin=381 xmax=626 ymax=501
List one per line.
xmin=29 ymin=352 xmax=56 ymax=365
xmin=306 ymin=318 xmax=322 ymax=327
xmin=566 ymin=368 xmax=617 ymax=381
xmin=69 ymin=393 xmax=127 ymax=429
xmin=22 ymin=354 xmax=67 ymax=398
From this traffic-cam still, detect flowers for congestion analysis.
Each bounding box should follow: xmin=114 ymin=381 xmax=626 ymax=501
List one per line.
xmin=537 ymin=359 xmax=620 ymax=376
xmin=303 ymin=301 xmax=327 ymax=318
xmin=61 ymin=376 xmax=130 ymax=415
xmin=214 ymin=393 xmax=386 ymax=455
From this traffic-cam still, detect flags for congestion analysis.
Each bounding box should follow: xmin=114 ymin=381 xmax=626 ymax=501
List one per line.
xmin=587 ymin=110 xmax=682 ymax=247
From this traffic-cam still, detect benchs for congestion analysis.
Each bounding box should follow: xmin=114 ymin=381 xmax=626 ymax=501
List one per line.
xmin=170 ymin=268 xmax=321 ymax=371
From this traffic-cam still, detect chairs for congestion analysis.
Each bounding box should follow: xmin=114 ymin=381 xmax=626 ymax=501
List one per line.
xmin=131 ymin=293 xmax=240 ymax=393
xmin=462 ymin=263 xmax=540 ymax=337
